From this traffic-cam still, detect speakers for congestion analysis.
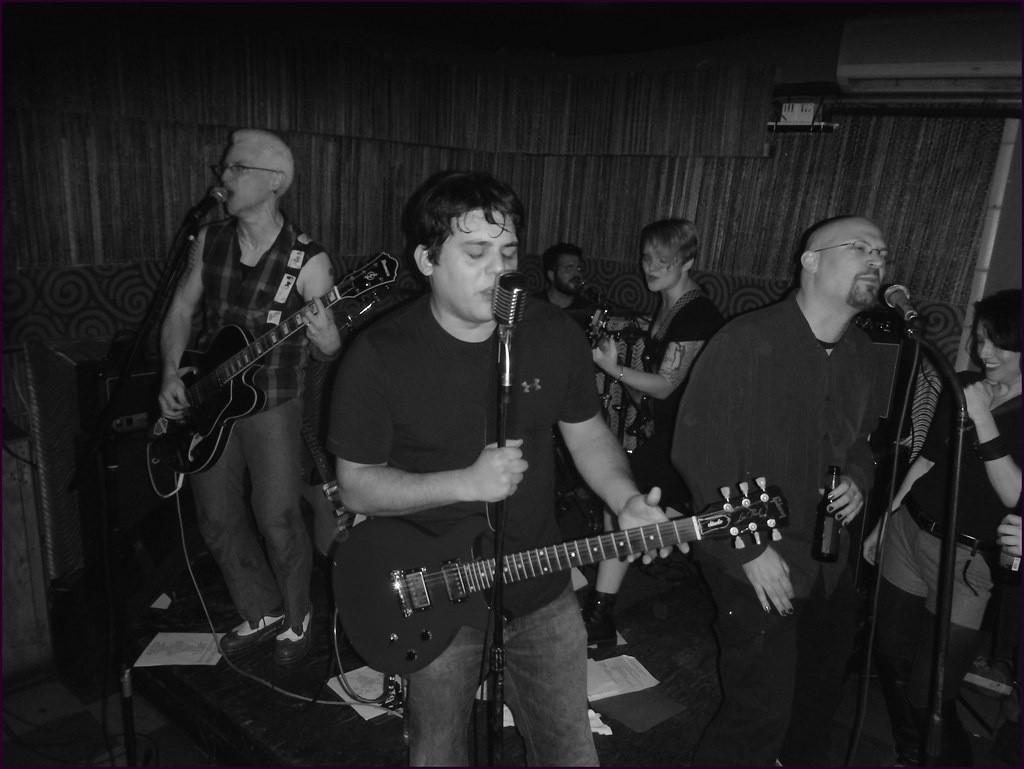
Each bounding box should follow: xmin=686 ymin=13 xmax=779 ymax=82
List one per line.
xmin=74 ymin=432 xmax=223 ymax=605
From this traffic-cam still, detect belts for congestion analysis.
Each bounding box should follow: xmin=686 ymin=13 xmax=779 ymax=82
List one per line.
xmin=904 ymin=495 xmax=985 ymax=551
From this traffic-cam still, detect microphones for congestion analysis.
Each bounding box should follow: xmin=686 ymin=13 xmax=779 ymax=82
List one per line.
xmin=185 ymin=186 xmax=227 ymax=223
xmin=884 ymin=285 xmax=918 ymax=320
xmin=491 ymin=270 xmax=528 ymax=325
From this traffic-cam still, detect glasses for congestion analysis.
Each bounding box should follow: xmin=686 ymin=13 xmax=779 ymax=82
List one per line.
xmin=814 ymin=241 xmax=889 ymax=260
xmin=560 ymin=265 xmax=582 ymax=271
xmin=215 ymin=165 xmax=284 ymax=174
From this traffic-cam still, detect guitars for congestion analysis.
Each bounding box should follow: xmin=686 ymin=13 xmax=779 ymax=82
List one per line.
xmin=329 ymin=475 xmax=795 ymax=674
xmin=588 ymin=308 xmax=653 ymax=452
xmin=148 ymin=250 xmax=398 ymax=469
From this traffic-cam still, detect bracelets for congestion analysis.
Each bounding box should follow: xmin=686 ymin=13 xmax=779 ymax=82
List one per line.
xmin=973 ymin=434 xmax=1013 ymax=464
xmin=617 ymin=365 xmax=624 ymax=380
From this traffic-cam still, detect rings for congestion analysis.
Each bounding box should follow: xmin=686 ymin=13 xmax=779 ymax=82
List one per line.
xmin=304 ymin=321 xmax=311 ymax=328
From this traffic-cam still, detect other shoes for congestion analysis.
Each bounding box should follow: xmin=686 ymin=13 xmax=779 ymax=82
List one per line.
xmin=273 ymin=601 xmax=314 ymax=664
xmin=581 ymin=587 xmax=618 ymax=649
xmin=218 ymin=617 xmax=285 ymax=654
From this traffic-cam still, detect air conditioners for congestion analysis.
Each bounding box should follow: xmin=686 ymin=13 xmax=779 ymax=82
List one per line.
xmin=835 ymin=12 xmax=1022 ymax=97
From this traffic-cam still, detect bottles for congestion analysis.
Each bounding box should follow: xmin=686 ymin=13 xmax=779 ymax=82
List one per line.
xmin=811 ymin=467 xmax=842 ymax=564
xmin=997 ymin=492 xmax=1023 ymax=586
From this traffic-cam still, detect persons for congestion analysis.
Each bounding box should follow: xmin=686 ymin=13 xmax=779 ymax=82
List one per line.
xmin=863 ymin=288 xmax=1024 ymax=769
xmin=327 ymin=170 xmax=691 ymax=769
xmin=160 ymin=133 xmax=398 ymax=664
xmin=530 ymin=219 xmax=727 ymax=646
xmin=996 ymin=513 xmax=1024 ymax=573
xmin=672 ymin=216 xmax=889 ymax=769
